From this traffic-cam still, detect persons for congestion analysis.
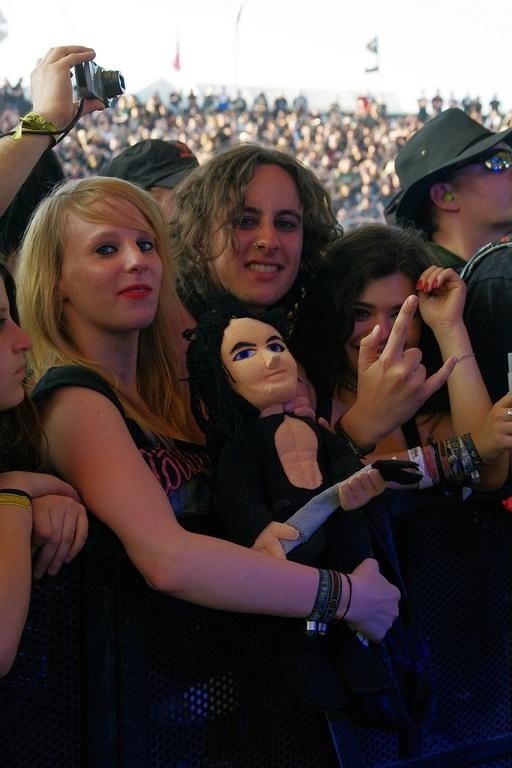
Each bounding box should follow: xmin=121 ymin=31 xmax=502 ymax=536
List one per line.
xmin=0 ymin=44 xmax=512 ymax=768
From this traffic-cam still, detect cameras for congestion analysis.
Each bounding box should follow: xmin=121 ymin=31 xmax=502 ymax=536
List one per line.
xmin=74 ymin=60 xmax=126 ymax=107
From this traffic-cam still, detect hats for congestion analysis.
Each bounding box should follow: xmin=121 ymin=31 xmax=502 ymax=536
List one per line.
xmin=99 ymin=138 xmax=201 ymax=194
xmin=383 ymin=108 xmax=512 ymax=225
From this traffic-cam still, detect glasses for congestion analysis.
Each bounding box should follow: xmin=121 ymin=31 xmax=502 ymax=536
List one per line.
xmin=443 ymin=147 xmax=510 ymax=178
